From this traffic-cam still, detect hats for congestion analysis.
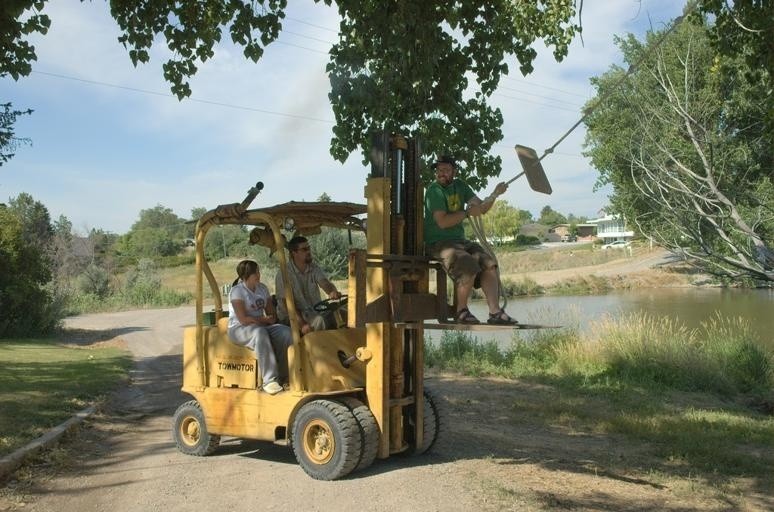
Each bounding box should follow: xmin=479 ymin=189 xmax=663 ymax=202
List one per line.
xmin=434 ymin=156 xmax=449 ymax=166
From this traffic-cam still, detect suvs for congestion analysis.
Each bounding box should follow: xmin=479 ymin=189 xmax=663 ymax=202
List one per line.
xmin=560 ymin=234 xmax=577 ymax=243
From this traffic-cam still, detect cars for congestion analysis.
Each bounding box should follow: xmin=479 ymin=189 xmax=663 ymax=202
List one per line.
xmin=600 ymin=240 xmax=629 ymax=250
xmin=174 ymin=238 xmax=197 ymax=258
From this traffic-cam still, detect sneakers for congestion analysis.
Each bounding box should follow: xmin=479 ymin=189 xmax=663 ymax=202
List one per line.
xmin=262 ymin=382 xmax=282 ymax=395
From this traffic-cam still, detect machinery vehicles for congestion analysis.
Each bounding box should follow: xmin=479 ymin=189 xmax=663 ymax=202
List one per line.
xmin=167 ymin=122 xmax=567 ymax=483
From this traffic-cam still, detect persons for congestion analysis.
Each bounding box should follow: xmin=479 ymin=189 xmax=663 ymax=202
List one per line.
xmin=424 ymin=154 xmax=518 ymax=327
xmin=274 ymin=236 xmax=348 ymax=336
xmin=227 ymin=260 xmax=291 ymax=394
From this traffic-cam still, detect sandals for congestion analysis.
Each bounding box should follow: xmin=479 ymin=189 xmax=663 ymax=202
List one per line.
xmin=453 ymin=308 xmax=479 ymax=324
xmin=488 ymin=309 xmax=519 ymax=324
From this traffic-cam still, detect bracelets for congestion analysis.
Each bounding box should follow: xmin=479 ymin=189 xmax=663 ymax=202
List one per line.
xmin=464 ymin=210 xmax=469 ymax=218
